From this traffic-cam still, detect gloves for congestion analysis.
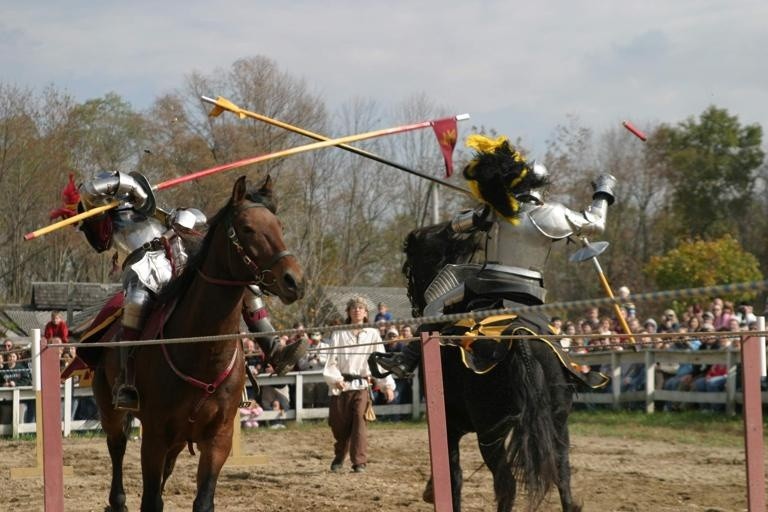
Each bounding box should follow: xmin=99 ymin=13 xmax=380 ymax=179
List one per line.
xmin=591 ymin=172 xmax=617 ymax=205
xmin=115 ymin=170 xmax=149 ymax=211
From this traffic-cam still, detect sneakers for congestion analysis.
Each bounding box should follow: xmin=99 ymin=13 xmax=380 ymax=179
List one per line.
xmin=353 ymin=467 xmax=363 ymax=472
xmin=331 ymin=455 xmax=347 ymax=471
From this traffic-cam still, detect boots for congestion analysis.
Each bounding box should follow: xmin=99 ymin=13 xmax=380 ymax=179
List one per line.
xmin=111 ymin=337 xmax=136 ymax=404
xmin=375 ymin=322 xmax=449 ymax=378
xmin=249 ymin=317 xmax=308 ymax=377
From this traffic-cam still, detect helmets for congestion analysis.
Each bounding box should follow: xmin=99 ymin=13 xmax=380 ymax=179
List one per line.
xmin=515 ymin=158 xmax=551 ymax=204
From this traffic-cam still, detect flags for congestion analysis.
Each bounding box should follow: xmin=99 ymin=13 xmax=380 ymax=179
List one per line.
xmin=432 ymin=117 xmax=458 ymax=180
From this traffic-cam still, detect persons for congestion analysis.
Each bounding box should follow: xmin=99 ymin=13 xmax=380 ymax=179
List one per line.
xmin=377 ymin=160 xmax=617 ymax=379
xmin=0 ymin=336 xmax=79 ymax=421
xmin=323 ymin=298 xmax=395 ymax=471
xmin=242 ymin=302 xmax=415 ymax=430
xmin=45 ymin=312 xmax=69 ymax=343
xmin=76 ymin=170 xmax=308 ymax=405
xmin=551 ymin=287 xmax=757 ymax=411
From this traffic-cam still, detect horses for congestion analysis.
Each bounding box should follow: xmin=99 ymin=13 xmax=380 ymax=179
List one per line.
xmin=403 ymin=220 xmax=582 ymax=512
xmin=91 ymin=174 xmax=306 ymax=512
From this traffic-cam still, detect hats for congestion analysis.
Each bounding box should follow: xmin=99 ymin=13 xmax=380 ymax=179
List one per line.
xmin=618 ymin=286 xmax=630 ymax=294
xmin=702 ymin=311 xmax=715 ymax=320
xmin=644 ymin=318 xmax=657 ymax=330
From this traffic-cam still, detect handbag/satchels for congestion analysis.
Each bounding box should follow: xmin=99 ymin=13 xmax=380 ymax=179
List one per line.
xmin=364 ymin=399 xmax=376 ymax=422
